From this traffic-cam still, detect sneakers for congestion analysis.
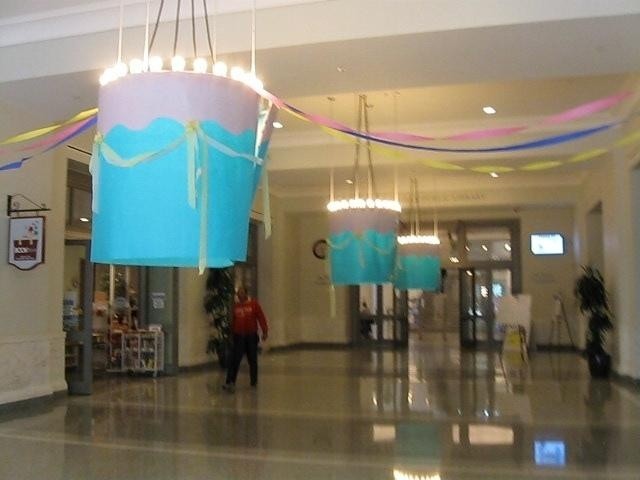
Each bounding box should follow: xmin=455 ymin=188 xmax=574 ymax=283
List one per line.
xmin=221 ymin=383 xmax=235 ymax=394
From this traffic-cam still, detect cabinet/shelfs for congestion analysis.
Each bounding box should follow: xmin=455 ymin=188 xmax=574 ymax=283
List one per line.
xmin=109 ymin=330 xmax=164 ymax=376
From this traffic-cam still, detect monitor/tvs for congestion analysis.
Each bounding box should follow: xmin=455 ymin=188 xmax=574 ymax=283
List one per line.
xmin=534 ymin=440 xmax=566 ymax=466
xmin=530 ymin=233 xmax=564 ymax=255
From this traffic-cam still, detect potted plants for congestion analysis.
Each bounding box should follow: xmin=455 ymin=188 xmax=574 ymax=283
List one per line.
xmin=202 ymin=269 xmax=235 ymax=368
xmin=574 ymin=263 xmax=615 ymax=378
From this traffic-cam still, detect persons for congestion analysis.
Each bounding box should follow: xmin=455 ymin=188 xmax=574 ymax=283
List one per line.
xmin=221 ymin=286 xmax=268 ymax=393
xmin=360 ymin=301 xmax=373 ymax=334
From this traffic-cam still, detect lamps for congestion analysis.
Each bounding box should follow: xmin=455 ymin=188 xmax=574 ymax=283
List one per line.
xmin=395 ymin=178 xmax=442 ymax=292
xmin=327 ymin=94 xmax=401 ymax=286
xmin=89 ymin=0 xmax=284 ymax=274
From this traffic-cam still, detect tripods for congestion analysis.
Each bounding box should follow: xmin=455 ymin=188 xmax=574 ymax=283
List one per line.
xmin=549 ymin=352 xmax=578 ymax=403
xmin=549 ymin=293 xmax=574 ymax=351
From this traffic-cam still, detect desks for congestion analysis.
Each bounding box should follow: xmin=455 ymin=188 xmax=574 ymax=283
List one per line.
xmin=65 ymin=341 xmax=83 ymax=369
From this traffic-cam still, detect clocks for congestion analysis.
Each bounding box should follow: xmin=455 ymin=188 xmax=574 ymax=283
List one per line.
xmin=313 ymin=240 xmax=327 ymax=259
